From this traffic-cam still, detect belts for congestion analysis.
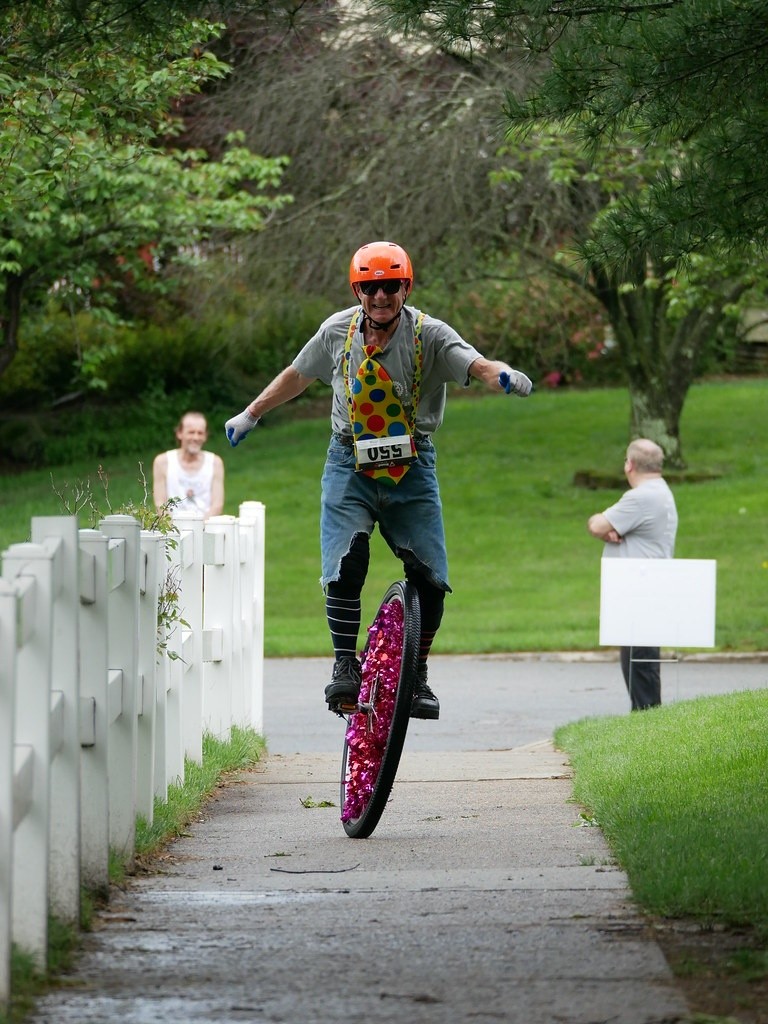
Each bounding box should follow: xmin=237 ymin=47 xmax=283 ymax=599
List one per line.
xmin=333 ymin=431 xmax=430 ymax=447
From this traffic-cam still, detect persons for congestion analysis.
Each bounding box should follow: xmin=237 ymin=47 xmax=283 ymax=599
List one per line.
xmin=153 ymin=412 xmax=224 ymax=532
xmin=588 ymin=438 xmax=679 ymax=711
xmin=226 ymin=242 xmax=529 ymax=719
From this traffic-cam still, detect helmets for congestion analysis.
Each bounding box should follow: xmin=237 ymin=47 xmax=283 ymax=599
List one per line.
xmin=350 ymin=241 xmax=414 ymax=296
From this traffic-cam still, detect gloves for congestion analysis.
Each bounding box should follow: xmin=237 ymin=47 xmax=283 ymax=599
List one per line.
xmin=224 ymin=408 xmax=261 ymax=447
xmin=498 ymin=369 xmax=532 ymax=397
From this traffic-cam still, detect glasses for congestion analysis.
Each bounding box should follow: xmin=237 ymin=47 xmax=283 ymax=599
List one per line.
xmin=358 ymin=279 xmax=402 ymax=296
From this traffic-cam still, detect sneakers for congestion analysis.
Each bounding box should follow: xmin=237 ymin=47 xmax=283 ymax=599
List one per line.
xmin=325 ymin=656 xmax=362 ymax=703
xmin=409 ymin=664 xmax=439 ymax=719
